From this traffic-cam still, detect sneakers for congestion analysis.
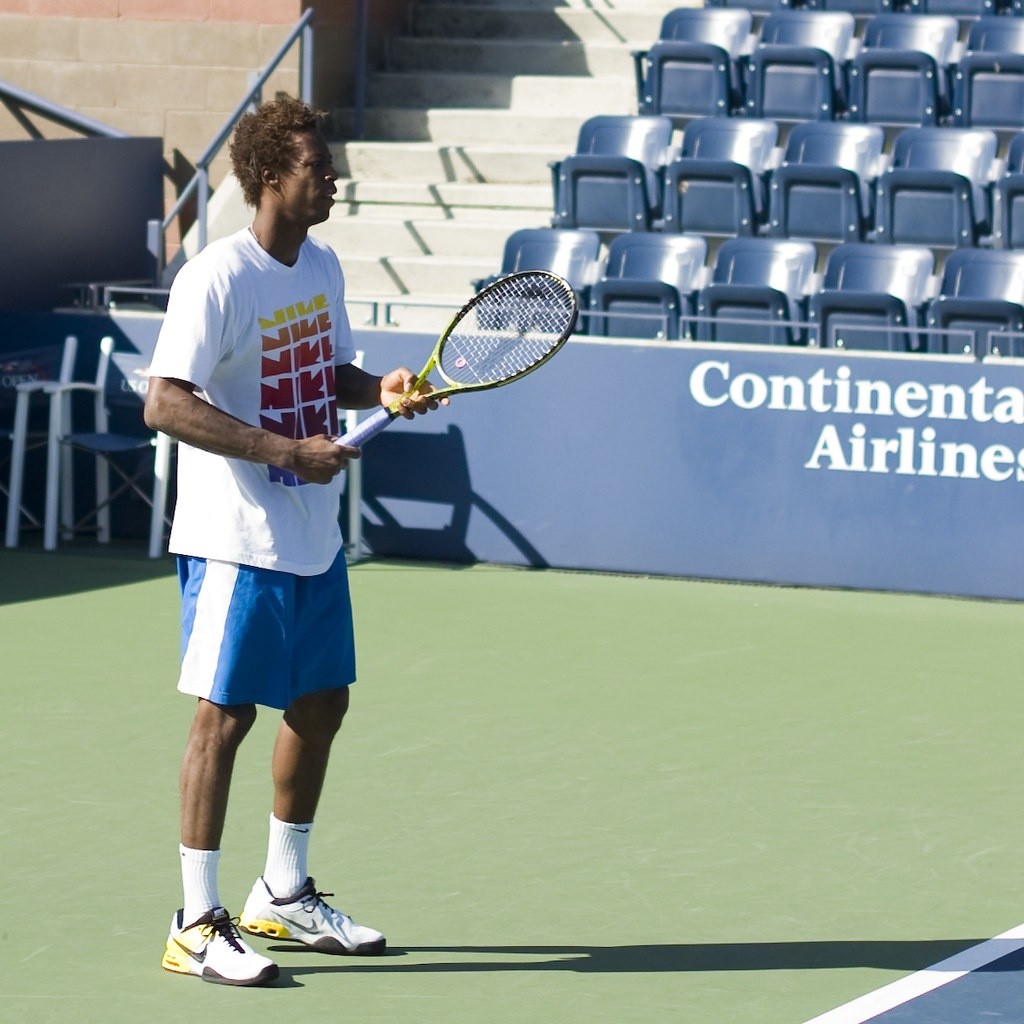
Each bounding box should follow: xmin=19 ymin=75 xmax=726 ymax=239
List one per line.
xmin=162 ymin=907 xmax=280 ymax=986
xmin=238 ymin=876 xmax=386 ymax=957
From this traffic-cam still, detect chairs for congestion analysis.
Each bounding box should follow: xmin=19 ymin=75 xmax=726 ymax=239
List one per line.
xmin=475 ymin=0 xmax=1024 ymax=357
xmin=0 ymin=334 xmax=175 ymax=559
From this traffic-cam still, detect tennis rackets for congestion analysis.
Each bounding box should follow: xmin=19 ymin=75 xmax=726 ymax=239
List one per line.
xmin=322 ymin=269 xmax=578 ymax=457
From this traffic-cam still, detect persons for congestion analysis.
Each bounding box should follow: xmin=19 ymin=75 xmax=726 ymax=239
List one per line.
xmin=142 ymin=90 xmax=448 ymax=987
